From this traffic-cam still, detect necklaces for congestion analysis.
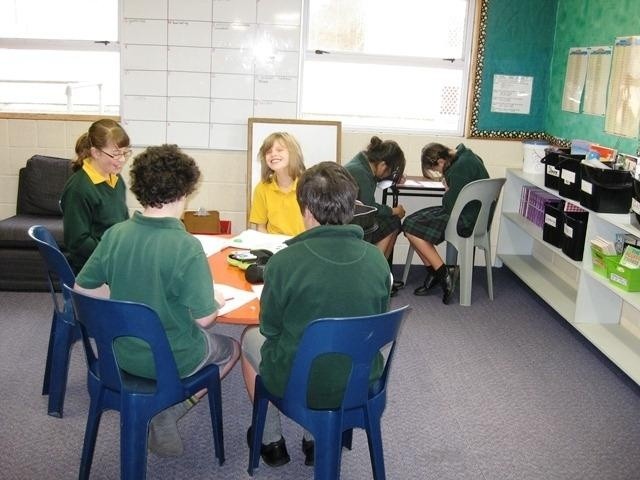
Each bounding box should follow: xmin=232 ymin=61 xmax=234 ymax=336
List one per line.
xmin=276 ymin=178 xmax=292 ymax=189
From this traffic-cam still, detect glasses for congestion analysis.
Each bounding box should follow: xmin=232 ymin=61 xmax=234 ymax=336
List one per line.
xmin=95 ymin=146 xmax=133 ymax=162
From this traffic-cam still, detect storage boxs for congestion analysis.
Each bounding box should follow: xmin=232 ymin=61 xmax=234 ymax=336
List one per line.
xmin=590 ymin=246 xmax=622 ymax=277
xmin=579 ymin=161 xmax=635 ymax=214
xmin=629 ymin=174 xmax=640 ymax=231
xmin=191 ymin=221 xmax=231 ymax=235
xmin=521 ymin=140 xmax=550 ymax=174
xmin=562 ymin=211 xmax=589 ymax=261
xmin=603 ymin=256 xmax=640 ymax=292
xmin=543 ymin=202 xmax=564 ymax=248
xmin=183 ymin=211 xmax=220 ymax=234
xmin=540 ymin=149 xmax=586 ymax=190
xmin=555 ymin=154 xmax=617 ymax=201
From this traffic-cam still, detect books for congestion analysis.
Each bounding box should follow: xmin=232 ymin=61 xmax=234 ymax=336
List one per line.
xmin=570 ymin=138 xmax=640 ymax=181
xmin=376 ymin=179 xmax=420 ymax=191
xmin=563 ymin=200 xmax=587 ymax=212
xmin=229 ymin=229 xmax=295 ymax=255
xmin=517 ymin=185 xmax=559 ymax=229
xmin=212 ymin=280 xmax=257 ymax=317
xmin=419 ymin=181 xmax=444 ymax=188
xmin=191 ymin=234 xmax=229 ymax=258
xmin=590 ymin=231 xmax=640 ymax=270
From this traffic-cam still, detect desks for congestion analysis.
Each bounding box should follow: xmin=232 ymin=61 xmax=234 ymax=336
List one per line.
xmin=382 ymin=175 xmax=476 ymax=274
xmin=193 ymin=234 xmax=264 ymax=325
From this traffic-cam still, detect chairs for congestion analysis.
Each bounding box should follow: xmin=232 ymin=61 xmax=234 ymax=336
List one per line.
xmin=401 ymin=178 xmax=507 ymax=306
xmin=28 ymin=225 xmax=94 ymax=418
xmin=247 ymin=304 xmax=413 ymax=480
xmin=63 ymin=282 xmax=225 ymax=480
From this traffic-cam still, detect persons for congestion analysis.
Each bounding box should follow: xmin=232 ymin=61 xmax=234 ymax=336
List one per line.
xmin=248 ymin=131 xmax=306 ymax=238
xmin=401 ymin=141 xmax=498 ymax=306
xmin=74 ymin=144 xmax=241 ymax=459
xmin=242 ymin=161 xmax=394 ymax=468
xmin=344 ymin=135 xmax=406 ymax=298
xmin=58 ymin=118 xmax=133 ymax=278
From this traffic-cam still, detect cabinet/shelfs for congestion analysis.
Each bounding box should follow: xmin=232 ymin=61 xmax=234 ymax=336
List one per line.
xmin=494 ymin=168 xmax=640 ymax=387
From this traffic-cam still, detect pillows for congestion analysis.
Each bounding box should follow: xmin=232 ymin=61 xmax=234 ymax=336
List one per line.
xmin=19 ymin=154 xmax=74 ymax=217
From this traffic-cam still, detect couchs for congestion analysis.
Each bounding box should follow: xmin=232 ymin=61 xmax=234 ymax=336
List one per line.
xmin=0 ymin=154 xmax=75 ymax=291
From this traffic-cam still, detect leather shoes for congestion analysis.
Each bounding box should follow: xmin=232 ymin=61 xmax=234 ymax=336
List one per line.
xmin=247 ymin=425 xmax=291 ymax=468
xmin=414 ymin=264 xmax=439 ymax=297
xmin=390 ymin=288 xmax=399 ymax=297
xmin=441 ymin=265 xmax=460 ymax=305
xmin=301 ymin=436 xmax=315 ymax=466
xmin=393 ymin=280 xmax=404 ymax=288
